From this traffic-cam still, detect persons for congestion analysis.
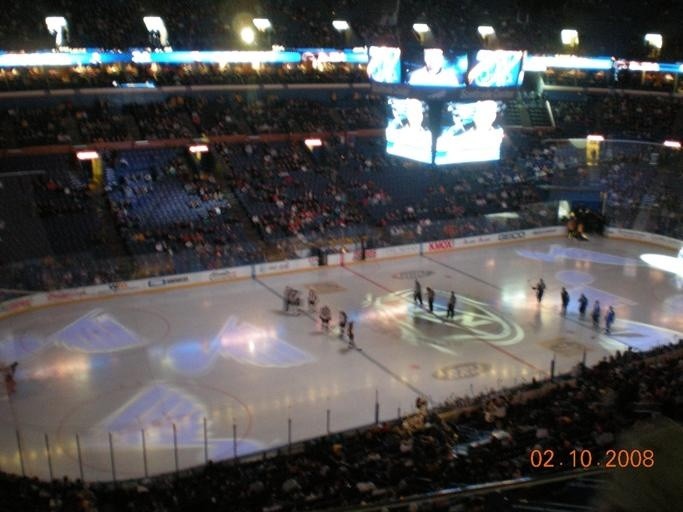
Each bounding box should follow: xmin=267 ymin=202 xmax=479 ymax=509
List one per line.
xmin=318 ymin=305 xmax=332 ymax=332
xmin=1 ymin=1 xmax=683 ymax=320
xmin=1 ymin=338 xmax=682 ymax=511
xmin=348 ymin=322 xmax=355 ymax=351
xmin=338 ymin=311 xmax=347 ymax=338
xmin=604 ymin=306 xmax=615 ymax=336
xmin=591 ymin=299 xmax=601 ymax=329
